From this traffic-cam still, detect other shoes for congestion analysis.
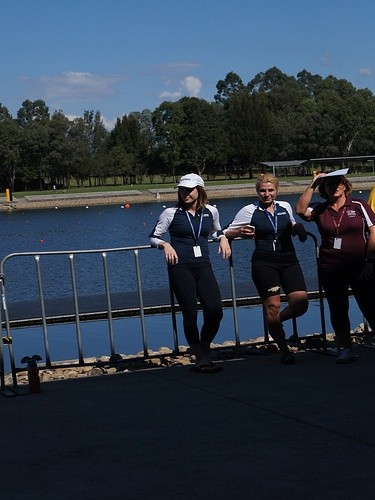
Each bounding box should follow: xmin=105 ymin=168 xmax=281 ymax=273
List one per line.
xmin=335 ymin=347 xmax=354 ymax=362
xmin=280 ymin=348 xmax=296 ymax=364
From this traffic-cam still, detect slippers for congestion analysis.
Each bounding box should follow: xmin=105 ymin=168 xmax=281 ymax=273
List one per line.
xmin=190 ymin=362 xmax=223 ymax=373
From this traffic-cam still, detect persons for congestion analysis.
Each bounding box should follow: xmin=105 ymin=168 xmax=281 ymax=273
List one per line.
xmin=294 ymin=171 xmax=375 ymax=362
xmin=222 ymin=173 xmax=309 ymax=364
xmin=147 ymin=173 xmax=232 ymax=371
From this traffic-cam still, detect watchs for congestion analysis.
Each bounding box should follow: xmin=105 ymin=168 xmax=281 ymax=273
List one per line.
xmin=363 ymin=255 xmax=375 ymax=263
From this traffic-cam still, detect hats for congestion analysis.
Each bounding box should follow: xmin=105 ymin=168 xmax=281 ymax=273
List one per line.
xmin=176 ymin=173 xmax=205 ymax=188
